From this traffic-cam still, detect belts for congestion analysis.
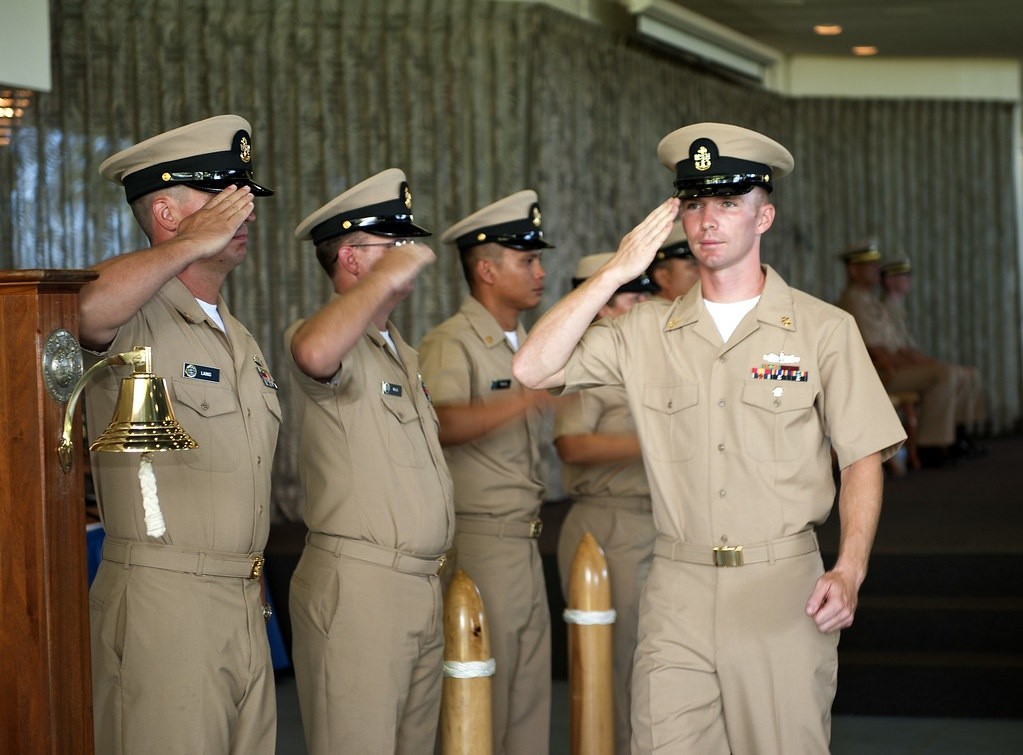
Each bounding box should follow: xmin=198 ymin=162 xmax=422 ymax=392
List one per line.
xmin=455 ymin=519 xmax=543 ymax=539
xmin=573 ymin=495 xmax=652 ymax=513
xmin=307 ymin=532 xmax=447 ymax=576
xmin=103 ymin=540 xmax=263 ymax=580
xmin=653 ymin=529 xmax=819 ymax=566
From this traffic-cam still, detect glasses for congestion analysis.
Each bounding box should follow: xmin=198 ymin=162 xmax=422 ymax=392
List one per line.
xmin=336 ymin=239 xmax=414 ymax=257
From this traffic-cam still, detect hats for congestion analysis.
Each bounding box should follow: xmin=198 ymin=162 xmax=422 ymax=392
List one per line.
xmin=831 ymin=238 xmax=882 ymax=268
xmin=657 ymin=123 xmax=794 ymax=199
xmin=98 ymin=114 xmax=275 ymax=203
xmin=878 ymin=255 xmax=912 ymax=277
xmin=645 ymin=221 xmax=695 ymax=264
xmin=439 ymin=189 xmax=556 ymax=250
xmin=294 ymin=168 xmax=432 ymax=246
xmin=571 ymin=251 xmax=660 ymax=292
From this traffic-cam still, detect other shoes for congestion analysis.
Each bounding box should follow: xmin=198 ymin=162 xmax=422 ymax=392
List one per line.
xmin=908 ymin=438 xmax=986 ymax=469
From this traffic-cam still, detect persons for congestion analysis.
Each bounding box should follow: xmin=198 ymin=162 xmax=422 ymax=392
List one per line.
xmin=511 ymin=121 xmax=904 ymax=754
xmin=77 ymin=111 xmax=559 ymax=755
xmin=831 ymin=235 xmax=986 ymax=469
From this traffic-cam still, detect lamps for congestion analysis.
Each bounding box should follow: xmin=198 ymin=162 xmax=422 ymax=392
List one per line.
xmin=43 ymin=331 xmax=197 ymax=470
